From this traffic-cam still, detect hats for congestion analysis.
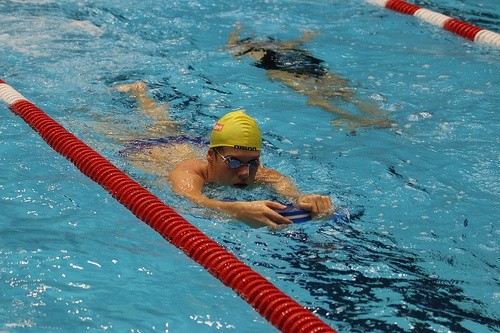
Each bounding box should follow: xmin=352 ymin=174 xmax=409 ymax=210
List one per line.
xmin=208 ymin=110 xmax=262 ymax=150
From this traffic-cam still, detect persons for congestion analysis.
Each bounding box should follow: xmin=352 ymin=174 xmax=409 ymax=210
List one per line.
xmin=216 ymin=29 xmax=395 ymax=131
xmin=83 ymin=79 xmax=334 ymax=233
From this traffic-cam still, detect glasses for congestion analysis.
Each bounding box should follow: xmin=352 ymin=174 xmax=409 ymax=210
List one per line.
xmin=213 ymin=147 xmax=260 ymax=169
